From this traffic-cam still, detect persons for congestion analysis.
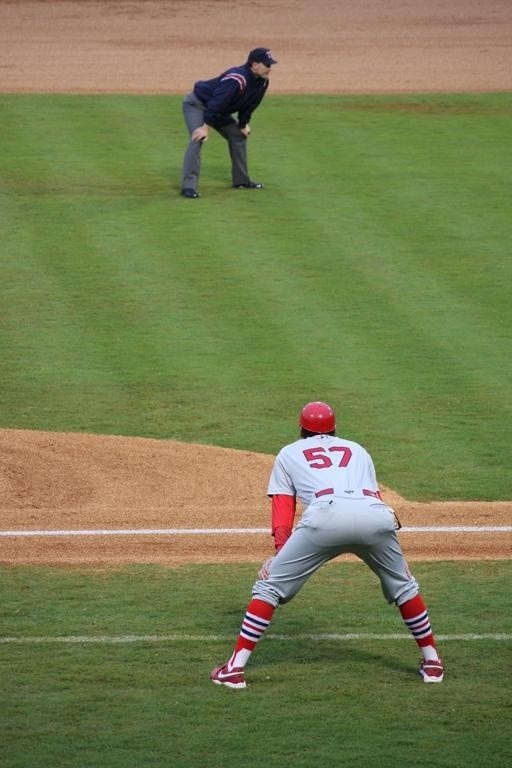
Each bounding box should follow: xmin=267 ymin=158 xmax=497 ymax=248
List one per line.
xmin=209 ymin=400 xmax=444 ymax=691
xmin=180 ymin=47 xmax=277 ymax=199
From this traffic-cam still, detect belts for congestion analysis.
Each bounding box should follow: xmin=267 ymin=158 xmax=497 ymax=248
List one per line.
xmin=315 ymin=488 xmax=380 ymax=499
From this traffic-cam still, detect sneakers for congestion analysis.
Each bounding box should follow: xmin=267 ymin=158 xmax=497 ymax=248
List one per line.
xmin=209 ymin=666 xmax=246 ymax=689
xmin=419 ymin=656 xmax=445 ymax=682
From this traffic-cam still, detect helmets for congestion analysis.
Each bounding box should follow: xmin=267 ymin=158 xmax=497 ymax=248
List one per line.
xmin=300 ymin=401 xmax=335 ymax=434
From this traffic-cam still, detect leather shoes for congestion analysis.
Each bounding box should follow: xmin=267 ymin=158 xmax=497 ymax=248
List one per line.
xmin=232 ymin=180 xmax=263 ymax=189
xmin=181 ymin=187 xmax=198 ymax=198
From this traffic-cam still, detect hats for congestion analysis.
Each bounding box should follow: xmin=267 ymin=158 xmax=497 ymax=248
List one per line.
xmin=248 ymin=47 xmax=278 ymax=65
